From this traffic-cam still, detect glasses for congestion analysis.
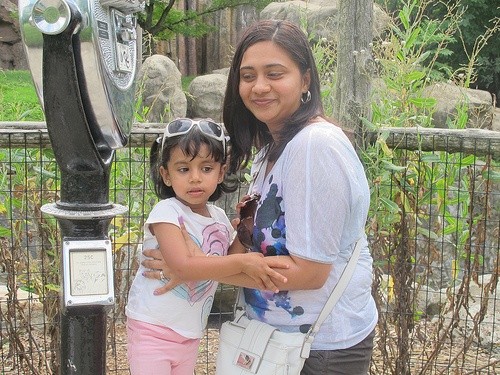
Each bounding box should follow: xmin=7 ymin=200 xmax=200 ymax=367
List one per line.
xmin=162 ymin=118 xmax=225 ymax=162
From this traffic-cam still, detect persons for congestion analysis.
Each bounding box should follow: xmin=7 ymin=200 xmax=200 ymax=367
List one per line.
xmin=141 ymin=19 xmax=379 ymax=375
xmin=125 ymin=118 xmax=290 ymax=375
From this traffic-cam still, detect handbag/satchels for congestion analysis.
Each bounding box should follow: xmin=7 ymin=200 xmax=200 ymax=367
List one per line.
xmin=216 ymin=309 xmax=314 ymax=375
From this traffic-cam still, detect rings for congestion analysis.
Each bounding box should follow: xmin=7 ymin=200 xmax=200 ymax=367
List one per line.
xmin=158 ymin=270 xmax=167 ymax=282
